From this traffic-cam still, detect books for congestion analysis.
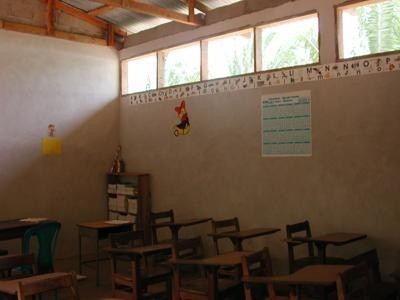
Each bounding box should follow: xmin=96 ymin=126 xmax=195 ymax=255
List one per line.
xmin=108 ymin=183 xmax=140 ymax=250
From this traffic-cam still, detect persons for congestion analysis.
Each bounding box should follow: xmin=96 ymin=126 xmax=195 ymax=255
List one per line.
xmin=42 ymin=124 xmax=61 ymax=154
xmin=110 ymin=145 xmax=125 ymax=174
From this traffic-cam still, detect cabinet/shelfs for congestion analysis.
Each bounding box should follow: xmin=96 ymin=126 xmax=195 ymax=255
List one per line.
xmin=106 ymin=172 xmax=150 ymax=245
